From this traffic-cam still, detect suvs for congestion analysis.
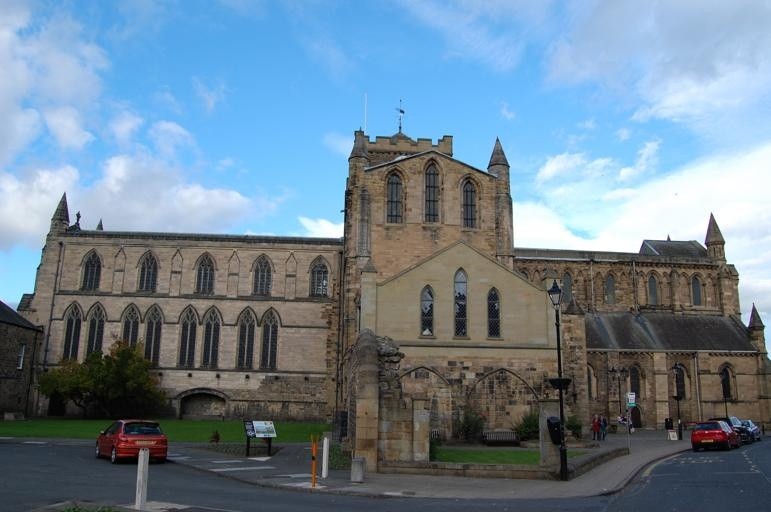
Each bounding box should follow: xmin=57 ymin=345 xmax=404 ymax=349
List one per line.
xmin=708 ymin=416 xmax=753 ymax=445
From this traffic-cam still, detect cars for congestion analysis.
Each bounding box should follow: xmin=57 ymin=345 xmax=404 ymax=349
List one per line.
xmin=740 ymin=420 xmax=762 ymax=443
xmin=692 ymin=420 xmax=741 ymax=451
xmin=95 ymin=421 xmax=169 ymax=464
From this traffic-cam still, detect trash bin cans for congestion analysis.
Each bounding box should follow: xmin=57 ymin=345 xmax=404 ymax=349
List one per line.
xmin=665 ymin=418 xmax=673 ymax=430
xmin=351 ymin=458 xmax=366 ymax=483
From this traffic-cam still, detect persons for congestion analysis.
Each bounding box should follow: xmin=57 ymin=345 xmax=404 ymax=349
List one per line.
xmin=598 ymin=415 xmax=608 ymax=441
xmin=618 ymin=416 xmax=635 ymax=434
xmin=591 ymin=413 xmax=599 ymax=440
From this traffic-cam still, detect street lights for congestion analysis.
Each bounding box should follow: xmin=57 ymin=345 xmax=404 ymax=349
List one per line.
xmin=671 ymin=363 xmax=683 ymax=441
xmin=548 ymin=279 xmax=569 ymax=479
xmin=719 ymin=371 xmax=728 ymax=417
